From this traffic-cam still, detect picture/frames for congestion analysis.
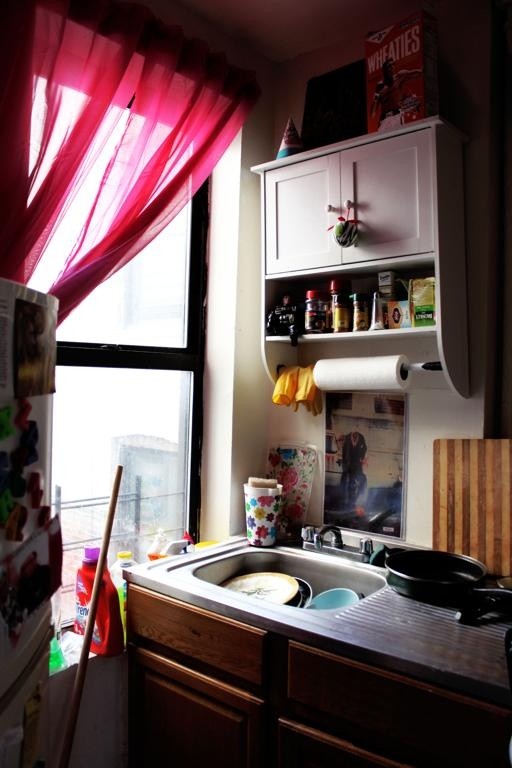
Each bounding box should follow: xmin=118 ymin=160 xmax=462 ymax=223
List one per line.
xmin=321 ymin=392 xmax=407 ymax=541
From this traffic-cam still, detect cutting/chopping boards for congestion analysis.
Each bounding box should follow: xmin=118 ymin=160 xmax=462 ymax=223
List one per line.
xmin=430 ymin=438 xmax=510 ymax=579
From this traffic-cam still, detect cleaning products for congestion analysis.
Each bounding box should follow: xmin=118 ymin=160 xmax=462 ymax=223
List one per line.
xmin=146 ymin=528 xmax=195 ymax=561
xmin=108 ymin=551 xmax=138 ymax=647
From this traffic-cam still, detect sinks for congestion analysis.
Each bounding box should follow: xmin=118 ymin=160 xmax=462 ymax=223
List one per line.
xmin=191 ymin=548 xmax=388 ymax=611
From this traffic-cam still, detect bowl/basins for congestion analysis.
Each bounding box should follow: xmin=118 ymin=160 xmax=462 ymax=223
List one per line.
xmin=308 ymin=587 xmax=360 ymax=611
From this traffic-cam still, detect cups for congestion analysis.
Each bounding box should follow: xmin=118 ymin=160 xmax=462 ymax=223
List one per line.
xmin=242 ymin=482 xmax=284 ymax=547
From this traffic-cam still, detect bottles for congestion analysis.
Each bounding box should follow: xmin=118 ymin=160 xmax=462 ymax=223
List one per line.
xmin=303 ymin=281 xmax=385 ymax=333
xmin=110 ymin=551 xmax=141 ymax=645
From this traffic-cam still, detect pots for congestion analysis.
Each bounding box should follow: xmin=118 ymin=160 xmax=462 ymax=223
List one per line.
xmin=384 ymin=546 xmax=511 ymax=607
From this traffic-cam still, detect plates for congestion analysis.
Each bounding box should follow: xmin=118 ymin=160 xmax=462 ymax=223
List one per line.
xmin=220 ymin=572 xmax=313 ymax=609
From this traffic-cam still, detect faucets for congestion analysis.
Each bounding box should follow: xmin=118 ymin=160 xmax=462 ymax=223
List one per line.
xmin=312 ymin=525 xmax=343 ymax=550
xmin=368 ymin=505 xmax=400 ymax=532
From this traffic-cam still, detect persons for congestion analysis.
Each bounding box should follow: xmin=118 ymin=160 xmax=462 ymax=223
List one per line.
xmin=19 ymin=552 xmax=50 ymax=616
xmin=339 ymin=421 xmax=367 ymax=524
xmin=370 ymin=58 xmax=422 ymax=131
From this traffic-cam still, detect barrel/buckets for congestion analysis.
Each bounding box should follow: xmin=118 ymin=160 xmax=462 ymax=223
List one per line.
xmin=72 ymin=547 xmax=126 ymax=658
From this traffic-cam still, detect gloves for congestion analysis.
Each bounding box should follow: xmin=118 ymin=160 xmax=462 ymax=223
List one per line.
xmin=272 ymin=364 xmax=323 ymax=417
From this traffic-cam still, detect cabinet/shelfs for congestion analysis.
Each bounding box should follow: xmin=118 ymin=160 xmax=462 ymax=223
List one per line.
xmin=250 ymin=113 xmax=464 ymax=278
xmin=125 ymin=581 xmax=272 ymax=768
xmin=271 ymin=630 xmax=512 ymax=768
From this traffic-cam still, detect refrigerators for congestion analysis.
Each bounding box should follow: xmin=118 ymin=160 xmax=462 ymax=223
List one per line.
xmin=0 ymin=277 xmax=62 ymax=767
